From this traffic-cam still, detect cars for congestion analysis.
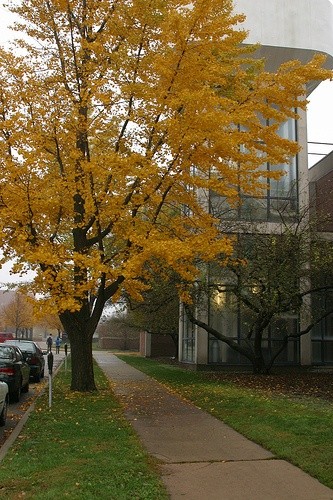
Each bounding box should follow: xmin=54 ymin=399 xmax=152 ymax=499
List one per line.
xmin=0 ymin=332 xmax=14 ymax=344
xmin=3 ymin=340 xmax=48 ymax=384
xmin=0 ymin=343 xmax=32 ymax=404
xmin=0 ymin=373 xmax=10 ymax=428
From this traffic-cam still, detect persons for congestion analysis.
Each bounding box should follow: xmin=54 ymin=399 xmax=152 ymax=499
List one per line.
xmin=55 ymin=338 xmax=60 ymax=354
xmin=46 ymin=334 xmax=53 ymax=353
